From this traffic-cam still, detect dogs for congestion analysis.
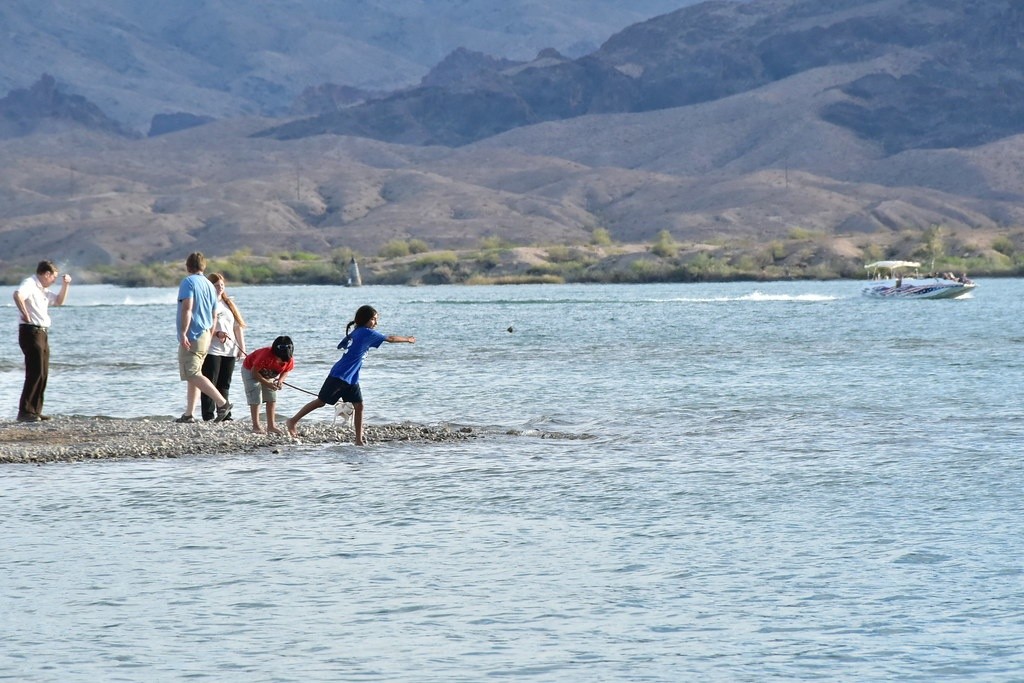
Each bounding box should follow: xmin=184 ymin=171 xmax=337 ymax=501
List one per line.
xmin=331 ymin=402 xmax=355 ymax=433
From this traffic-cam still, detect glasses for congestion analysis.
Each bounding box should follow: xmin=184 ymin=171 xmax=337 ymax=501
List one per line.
xmin=277 ymin=344 xmax=294 ymax=350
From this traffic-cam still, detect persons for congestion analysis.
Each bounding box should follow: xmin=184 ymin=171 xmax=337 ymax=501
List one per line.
xmin=286 ymin=305 xmax=416 ymax=446
xmin=872 ymin=269 xmax=974 ymax=285
xmin=172 ymin=251 xmax=244 ymax=423
xmin=241 ymin=336 xmax=294 ymax=434
xmin=13 ymin=261 xmax=72 ymax=422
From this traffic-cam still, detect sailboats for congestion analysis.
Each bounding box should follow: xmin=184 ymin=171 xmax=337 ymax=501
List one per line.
xmin=346 ymin=257 xmax=363 ymax=287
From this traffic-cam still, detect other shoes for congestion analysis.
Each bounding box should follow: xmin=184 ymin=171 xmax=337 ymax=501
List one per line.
xmin=17 ymin=414 xmax=51 ymax=421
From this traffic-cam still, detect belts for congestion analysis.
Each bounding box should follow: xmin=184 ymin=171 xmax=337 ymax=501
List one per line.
xmin=19 ymin=325 xmax=47 ymax=332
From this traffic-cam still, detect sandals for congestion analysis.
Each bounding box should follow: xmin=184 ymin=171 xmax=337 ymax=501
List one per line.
xmin=214 ymin=401 xmax=233 ymax=423
xmin=172 ymin=413 xmax=195 ymax=423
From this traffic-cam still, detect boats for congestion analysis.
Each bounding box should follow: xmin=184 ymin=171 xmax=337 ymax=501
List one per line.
xmin=861 ymin=260 xmax=979 ymax=299
